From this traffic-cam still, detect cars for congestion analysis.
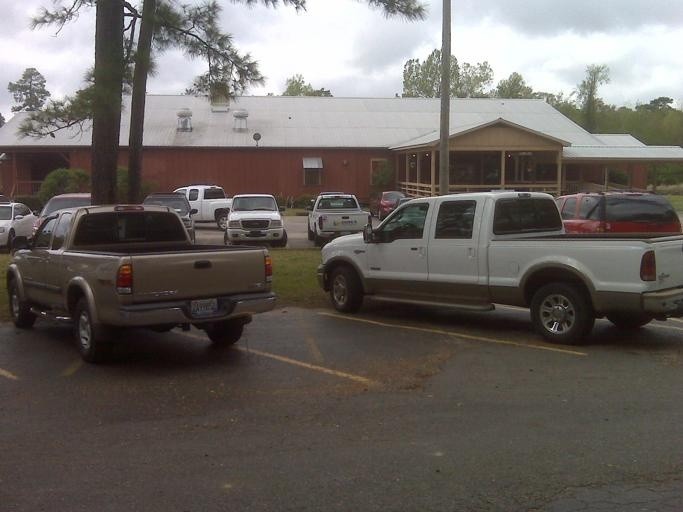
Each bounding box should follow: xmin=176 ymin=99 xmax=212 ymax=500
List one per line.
xmin=27 ymin=193 xmax=93 ymax=247
xmin=0 ymin=202 xmax=41 ymax=248
xmin=369 ymin=190 xmax=405 ymax=222
xmin=141 ymin=191 xmax=198 ymax=244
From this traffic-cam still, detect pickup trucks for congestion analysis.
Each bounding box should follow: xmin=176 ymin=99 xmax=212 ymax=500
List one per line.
xmin=314 ymin=186 xmax=683 ymax=349
xmin=172 ymin=184 xmax=232 ymax=231
xmin=306 ymin=192 xmax=373 ymax=246
xmin=4 ymin=202 xmax=278 ymax=361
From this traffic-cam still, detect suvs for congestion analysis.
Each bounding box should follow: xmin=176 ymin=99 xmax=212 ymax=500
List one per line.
xmin=555 ymin=190 xmax=682 ymax=237
xmin=224 ymin=193 xmax=288 ymax=248
